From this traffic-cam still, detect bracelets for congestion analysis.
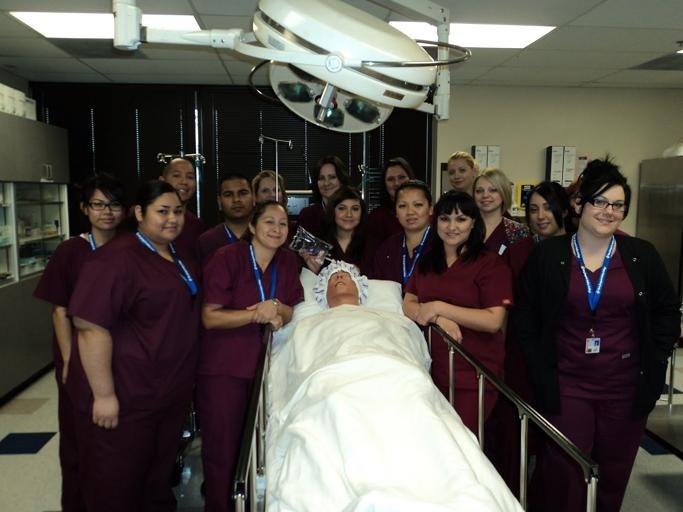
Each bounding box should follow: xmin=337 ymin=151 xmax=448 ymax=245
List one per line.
xmin=435 ymin=314 xmax=440 ymax=324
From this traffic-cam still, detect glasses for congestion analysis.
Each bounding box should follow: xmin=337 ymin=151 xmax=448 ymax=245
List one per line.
xmin=86 ymin=199 xmax=125 ymax=212
xmin=587 ymin=198 xmax=627 ymax=213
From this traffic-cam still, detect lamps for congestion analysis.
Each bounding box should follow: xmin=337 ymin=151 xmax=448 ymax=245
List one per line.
xmin=111 ymin=0 xmax=452 ymax=135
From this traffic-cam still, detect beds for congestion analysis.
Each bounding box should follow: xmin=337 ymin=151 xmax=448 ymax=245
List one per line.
xmin=230 ymin=275 xmax=600 ymax=511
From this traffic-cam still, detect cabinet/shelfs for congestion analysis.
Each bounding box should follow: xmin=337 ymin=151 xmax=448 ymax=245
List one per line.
xmin=14 ymin=182 xmax=70 ymax=279
xmin=0 ymin=181 xmax=18 ymax=287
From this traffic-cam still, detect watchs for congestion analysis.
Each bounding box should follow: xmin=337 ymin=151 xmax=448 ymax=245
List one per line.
xmin=271 ymin=298 xmax=279 ymax=312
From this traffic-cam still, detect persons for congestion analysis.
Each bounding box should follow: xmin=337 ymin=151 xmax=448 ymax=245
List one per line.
xmin=253 ymin=171 xmax=299 ymax=235
xmin=403 ymin=192 xmax=511 ymax=435
xmin=504 ymin=158 xmax=673 ymax=512
xmin=448 ymin=153 xmax=478 ymax=192
xmin=197 ymin=172 xmax=256 ymax=273
xmin=299 ymin=156 xmax=354 ymax=229
xmin=156 ymin=156 xmax=207 ymax=265
xmin=197 ymin=198 xmax=304 ymax=512
xmin=528 ymin=181 xmax=573 ymax=239
xmin=472 ymin=168 xmax=532 ymax=273
xmin=311 ymin=185 xmax=377 ymax=261
xmin=33 ymin=175 xmax=133 ymax=512
xmin=66 ymin=181 xmax=201 ymax=512
xmin=370 ymin=180 xmax=437 ymax=299
xmin=365 ymin=157 xmax=434 ymax=239
